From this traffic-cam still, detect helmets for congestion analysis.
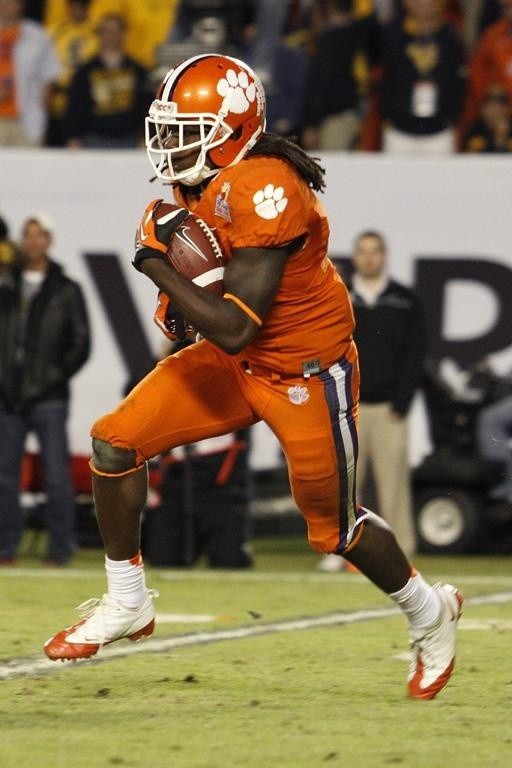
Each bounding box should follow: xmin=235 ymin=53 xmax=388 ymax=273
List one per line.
xmin=142 ymin=54 xmax=266 ymax=186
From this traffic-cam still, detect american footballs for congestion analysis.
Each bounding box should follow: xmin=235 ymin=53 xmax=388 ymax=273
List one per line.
xmin=154 ymin=200 xmax=226 ymax=302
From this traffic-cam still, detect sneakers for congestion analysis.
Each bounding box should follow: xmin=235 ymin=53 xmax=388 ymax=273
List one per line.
xmin=408 ymin=582 xmax=462 ymax=701
xmin=43 ymin=588 xmax=159 ymax=659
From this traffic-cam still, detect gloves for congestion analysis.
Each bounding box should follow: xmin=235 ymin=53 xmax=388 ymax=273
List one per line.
xmin=154 ymin=287 xmax=196 ymax=341
xmin=132 ymin=199 xmax=187 ymax=269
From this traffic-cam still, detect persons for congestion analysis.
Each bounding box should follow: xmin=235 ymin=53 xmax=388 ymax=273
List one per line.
xmin=315 ymin=231 xmax=424 ymax=576
xmin=246 ymin=1 xmax=511 ymax=155
xmin=1 ymin=0 xmax=180 ymax=148
xmin=43 ymin=50 xmax=466 ymax=699
xmin=0 ymin=212 xmax=91 ymax=569
